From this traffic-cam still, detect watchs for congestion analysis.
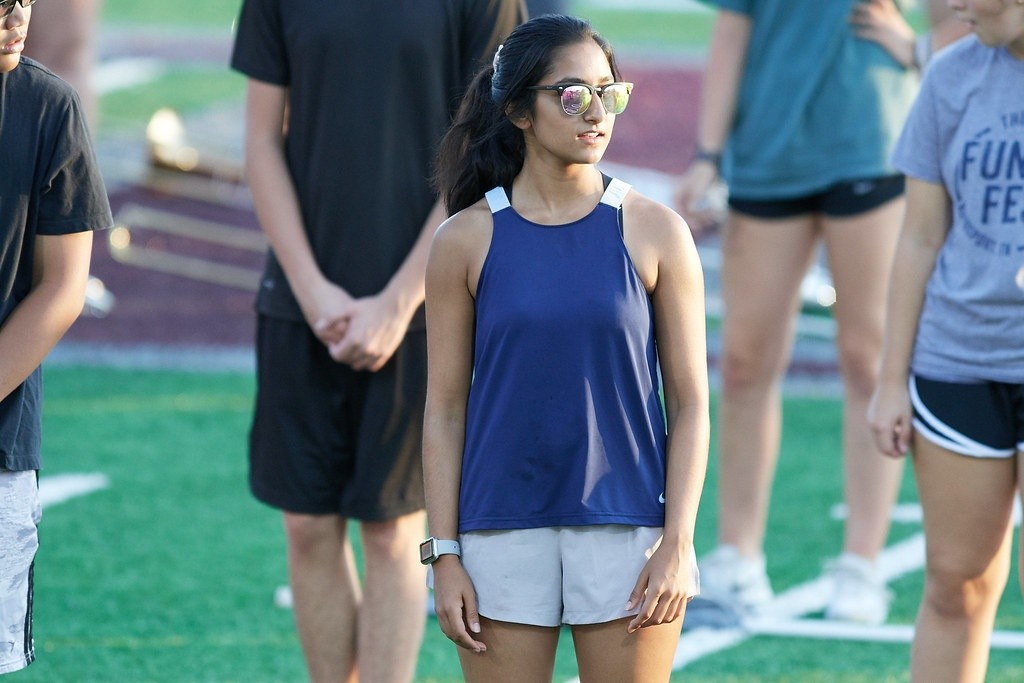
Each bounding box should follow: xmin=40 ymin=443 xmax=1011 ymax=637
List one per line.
xmin=421 ymin=537 xmax=461 ymax=566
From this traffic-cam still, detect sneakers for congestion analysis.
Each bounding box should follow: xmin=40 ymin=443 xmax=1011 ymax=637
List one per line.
xmin=822 ymin=556 xmax=889 ymax=627
xmin=684 ymin=550 xmax=774 ymax=619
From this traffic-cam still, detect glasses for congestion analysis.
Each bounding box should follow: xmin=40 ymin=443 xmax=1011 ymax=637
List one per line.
xmin=518 ymin=83 xmax=635 ymax=116
xmin=0 ymin=0 xmax=36 ymax=19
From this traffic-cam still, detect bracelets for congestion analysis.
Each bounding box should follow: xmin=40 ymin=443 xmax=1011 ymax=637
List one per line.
xmin=693 ymin=150 xmax=721 ymax=169
xmin=907 ymin=20 xmax=935 ymax=75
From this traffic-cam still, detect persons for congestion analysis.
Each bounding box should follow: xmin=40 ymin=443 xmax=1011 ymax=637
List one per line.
xmin=220 ymin=0 xmax=532 ymax=683
xmin=0 ymin=2 xmax=120 ymax=683
xmin=667 ymin=0 xmax=937 ymax=645
xmin=417 ymin=10 xmax=710 ymax=681
xmin=866 ymin=0 xmax=1023 ymax=683
